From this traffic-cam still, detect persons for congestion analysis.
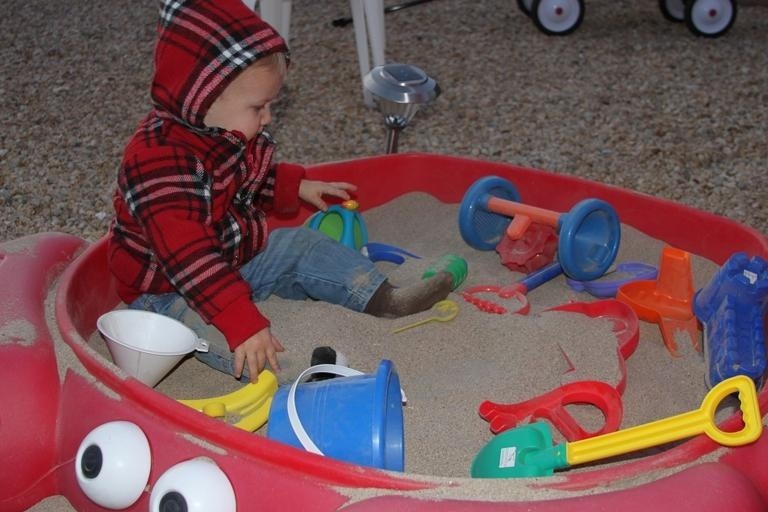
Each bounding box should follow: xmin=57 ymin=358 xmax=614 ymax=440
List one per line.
xmin=107 ymin=0 xmax=455 ymax=389
